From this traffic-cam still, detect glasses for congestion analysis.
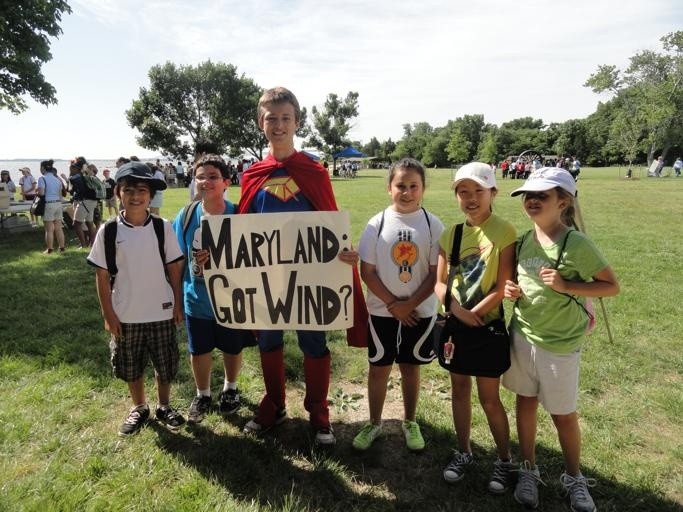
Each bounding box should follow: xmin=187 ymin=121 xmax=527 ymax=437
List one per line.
xmin=194 ymin=175 xmax=225 ymax=184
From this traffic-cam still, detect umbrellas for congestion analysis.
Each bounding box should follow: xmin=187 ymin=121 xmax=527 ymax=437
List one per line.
xmin=297 ymin=149 xmax=320 ymax=160
xmin=335 ymin=146 xmax=369 ymax=157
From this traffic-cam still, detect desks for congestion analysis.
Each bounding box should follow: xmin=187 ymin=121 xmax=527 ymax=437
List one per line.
xmin=10 ymin=198 xmax=71 ymax=233
xmin=0 ymin=202 xmax=38 ymax=230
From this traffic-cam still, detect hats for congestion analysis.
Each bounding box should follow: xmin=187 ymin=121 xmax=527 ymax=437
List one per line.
xmin=113 ymin=160 xmax=167 ymax=192
xmin=70 ymin=160 xmax=83 ymax=170
xmin=510 ymin=166 xmax=577 ymax=198
xmin=450 ymin=161 xmax=498 ymax=191
xmin=19 ymin=167 xmax=31 ymax=173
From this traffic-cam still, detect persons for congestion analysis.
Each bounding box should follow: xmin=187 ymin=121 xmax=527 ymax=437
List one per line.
xmin=0 ymin=140 xmax=257 ymax=256
xmin=194 ymin=88 xmax=358 ymax=448
xmin=655 ymin=156 xmax=664 ymax=177
xmin=86 ymin=161 xmax=185 ymax=438
xmin=672 ymin=157 xmax=683 ymax=177
xmin=493 ymin=156 xmax=581 ymax=182
xmin=504 ymin=167 xmax=620 ymax=512
xmin=171 ymin=154 xmax=259 ymax=422
xmin=434 ymin=161 xmax=516 ymax=496
xmin=324 ymin=160 xmax=390 ymax=178
xmin=351 ymin=157 xmax=446 ymax=452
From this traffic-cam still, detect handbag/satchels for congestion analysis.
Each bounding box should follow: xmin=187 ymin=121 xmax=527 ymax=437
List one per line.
xmin=437 ymin=314 xmax=511 ymax=378
xmin=29 ymin=192 xmax=46 ymax=216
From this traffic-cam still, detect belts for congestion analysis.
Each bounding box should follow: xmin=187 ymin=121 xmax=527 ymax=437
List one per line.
xmin=44 ymin=199 xmax=62 ymax=204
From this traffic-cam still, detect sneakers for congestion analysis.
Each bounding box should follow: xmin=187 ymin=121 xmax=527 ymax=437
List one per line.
xmin=352 ymin=419 xmax=382 ymax=451
xmin=401 ymin=420 xmax=426 ymax=451
xmin=562 ymin=470 xmax=597 ymax=512
xmin=56 ymin=247 xmax=65 ymax=254
xmin=242 ymin=405 xmax=288 ymax=436
xmin=154 ymin=402 xmax=187 ymax=431
xmin=489 ymin=455 xmax=516 ymax=494
xmin=443 ymin=448 xmax=473 ymax=483
xmin=315 ymin=423 xmax=335 ymax=445
xmin=116 ymin=403 xmax=154 ymax=438
xmin=218 ymin=387 xmax=241 ymax=416
xmin=43 ymin=248 xmax=54 ymax=255
xmin=187 ymin=394 xmax=214 ymax=424
xmin=514 ymin=462 xmax=541 ymax=509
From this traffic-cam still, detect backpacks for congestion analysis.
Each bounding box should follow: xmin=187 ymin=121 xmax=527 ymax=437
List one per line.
xmin=511 ymin=228 xmax=596 ymax=334
xmin=78 ymin=172 xmax=107 ymax=200
